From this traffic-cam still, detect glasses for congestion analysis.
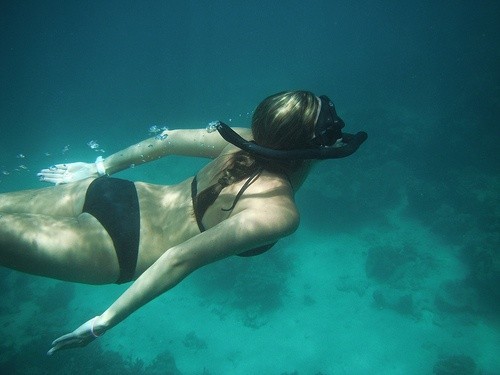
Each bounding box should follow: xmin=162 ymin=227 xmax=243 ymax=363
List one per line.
xmin=310 ymin=94 xmax=345 ymax=147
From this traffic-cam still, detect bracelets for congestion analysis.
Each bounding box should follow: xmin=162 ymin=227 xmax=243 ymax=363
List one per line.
xmin=95 ymin=155 xmax=109 ymax=178
xmin=89 ymin=315 xmax=104 ymax=339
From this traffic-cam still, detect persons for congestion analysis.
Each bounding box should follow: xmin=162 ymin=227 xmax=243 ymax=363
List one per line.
xmin=0 ymin=88 xmax=346 ymax=355
